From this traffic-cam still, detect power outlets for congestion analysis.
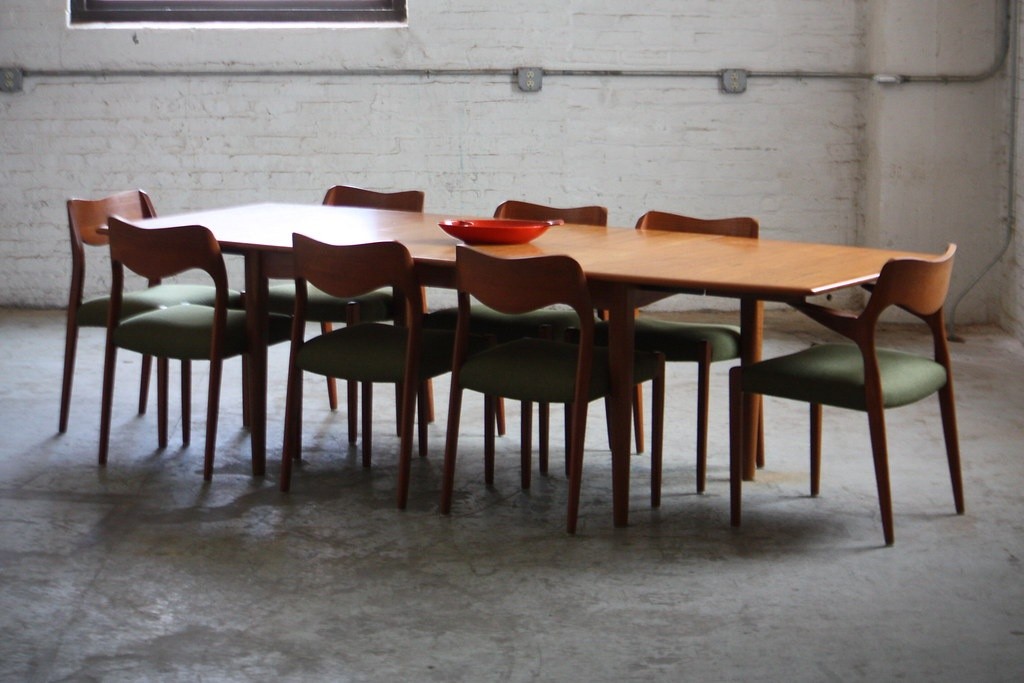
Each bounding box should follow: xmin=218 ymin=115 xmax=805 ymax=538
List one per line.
xmin=722 ymin=68 xmax=747 ymax=93
xmin=0 ymin=67 xmax=23 ymax=93
xmin=517 ymin=67 xmax=542 ymax=92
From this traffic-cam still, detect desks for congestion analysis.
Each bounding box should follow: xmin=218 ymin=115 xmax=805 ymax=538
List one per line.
xmin=95 ymin=201 xmax=944 ymax=482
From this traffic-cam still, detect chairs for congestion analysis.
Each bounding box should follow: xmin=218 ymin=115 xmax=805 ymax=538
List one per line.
xmin=281 ymin=231 xmax=499 ymax=511
xmin=58 ymin=190 xmax=250 ymax=433
xmin=593 ymin=210 xmax=765 ymax=492
xmin=98 ymin=213 xmax=304 ymax=481
xmin=414 ymin=200 xmax=608 ymax=479
xmin=240 ymin=185 xmax=435 ymax=443
xmin=729 ymin=242 xmax=965 ymax=547
xmin=440 ymin=243 xmax=667 ymax=538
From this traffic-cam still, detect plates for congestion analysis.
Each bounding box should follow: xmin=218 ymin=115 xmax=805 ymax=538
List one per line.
xmin=437 ymin=220 xmax=564 ymax=245
